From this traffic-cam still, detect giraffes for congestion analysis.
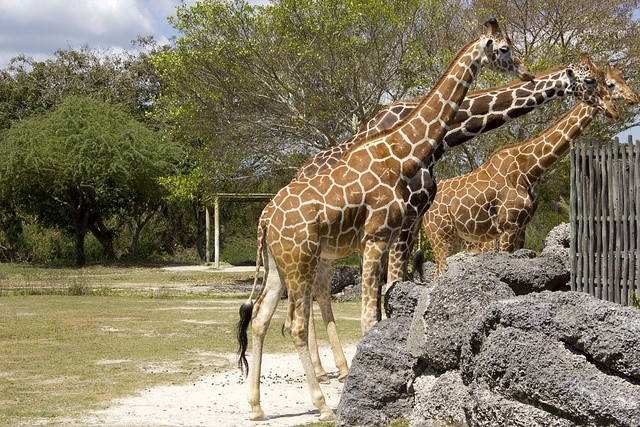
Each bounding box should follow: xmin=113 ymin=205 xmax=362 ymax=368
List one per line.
xmin=292 ymin=50 xmax=620 ymax=384
xmin=234 ymin=18 xmax=536 ymax=421
xmin=412 ymin=61 xmax=640 ymax=283
